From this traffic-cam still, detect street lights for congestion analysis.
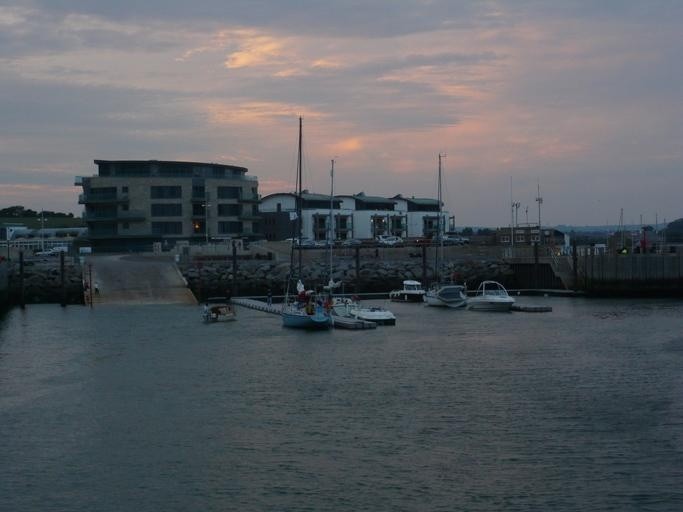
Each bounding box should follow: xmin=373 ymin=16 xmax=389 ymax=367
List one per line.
xmin=512 ymin=199 xmax=520 ymax=226
xmin=36 ymin=207 xmax=49 ymax=253
xmin=201 ymin=193 xmax=212 ymax=245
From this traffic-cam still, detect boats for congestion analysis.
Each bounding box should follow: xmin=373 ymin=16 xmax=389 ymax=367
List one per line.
xmin=202 ymin=305 xmax=236 ymax=322
xmin=421 ymin=282 xmax=466 ymax=308
xmin=466 ymin=280 xmax=516 ymax=312
xmin=349 ymin=306 xmax=397 ymax=326
xmin=388 ymin=279 xmax=426 ymax=303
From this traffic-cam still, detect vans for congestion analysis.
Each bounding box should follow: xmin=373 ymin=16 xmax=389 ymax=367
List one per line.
xmin=376 ymin=234 xmax=389 ymax=241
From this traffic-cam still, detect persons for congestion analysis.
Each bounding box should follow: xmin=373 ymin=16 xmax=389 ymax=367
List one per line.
xmin=202 ymin=304 xmax=211 ymax=313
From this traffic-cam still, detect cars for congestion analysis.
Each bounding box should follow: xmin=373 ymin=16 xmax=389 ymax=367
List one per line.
xmin=287 ymin=236 xmax=335 ymax=249
xmin=383 ymin=236 xmax=405 ymax=248
xmin=341 ymin=238 xmax=362 ymax=249
xmin=430 ymin=231 xmax=470 ymax=246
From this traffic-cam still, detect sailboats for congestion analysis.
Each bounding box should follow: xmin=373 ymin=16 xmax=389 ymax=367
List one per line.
xmin=423 ymin=152 xmax=467 ymax=310
xmin=280 ymin=116 xmax=334 ymax=329
